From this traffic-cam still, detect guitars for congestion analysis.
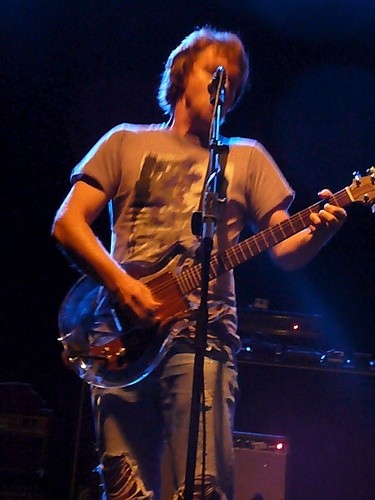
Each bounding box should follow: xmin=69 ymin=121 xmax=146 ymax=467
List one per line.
xmin=55 ymin=165 xmax=375 ymax=390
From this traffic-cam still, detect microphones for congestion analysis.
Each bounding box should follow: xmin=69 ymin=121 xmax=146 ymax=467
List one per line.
xmin=207 ymin=65 xmax=226 ymax=94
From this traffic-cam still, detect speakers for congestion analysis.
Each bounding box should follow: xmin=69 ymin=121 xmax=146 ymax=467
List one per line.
xmin=231 ymin=430 xmax=286 ymax=500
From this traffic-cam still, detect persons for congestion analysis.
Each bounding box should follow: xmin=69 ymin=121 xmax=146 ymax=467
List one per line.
xmin=51 ymin=25 xmax=347 ymax=500
